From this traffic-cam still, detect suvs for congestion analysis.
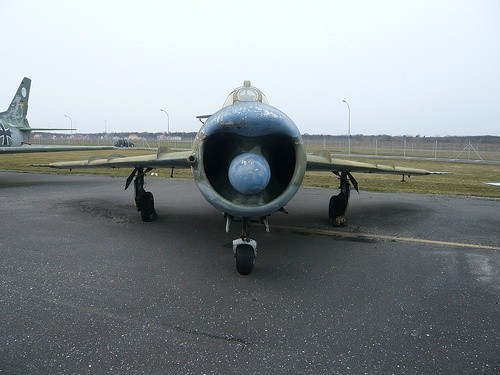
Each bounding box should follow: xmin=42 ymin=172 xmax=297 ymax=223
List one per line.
xmin=114 ymin=139 xmax=134 ymax=148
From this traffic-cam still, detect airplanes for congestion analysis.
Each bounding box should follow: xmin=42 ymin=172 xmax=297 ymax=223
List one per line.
xmin=0 ymin=77 xmax=122 ymax=152
xmin=26 ymin=80 xmax=452 ymax=277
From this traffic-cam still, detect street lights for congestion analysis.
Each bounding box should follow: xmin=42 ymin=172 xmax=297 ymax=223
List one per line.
xmin=63 ymin=113 xmax=72 ymax=138
xmin=341 ymin=98 xmax=351 ymax=137
xmin=160 ymin=109 xmax=170 ymax=140
